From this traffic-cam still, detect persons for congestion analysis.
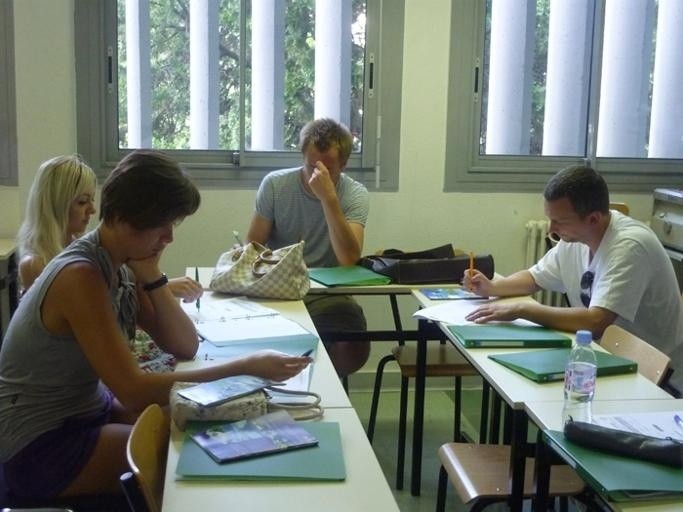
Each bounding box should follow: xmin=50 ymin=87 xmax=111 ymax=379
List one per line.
xmin=18 ymin=153 xmax=203 ymax=377
xmin=461 ymin=163 xmax=682 ymax=398
xmin=230 ymin=116 xmax=371 ymax=378
xmin=0 ymin=148 xmax=313 ymax=512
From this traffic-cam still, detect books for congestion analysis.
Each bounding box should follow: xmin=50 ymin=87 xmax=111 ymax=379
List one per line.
xmin=420 ymin=286 xmax=488 ymax=300
xmin=180 ymin=294 xmax=308 ymax=347
xmin=186 ymin=408 xmax=320 ymax=465
xmin=176 ymin=373 xmax=275 ymax=406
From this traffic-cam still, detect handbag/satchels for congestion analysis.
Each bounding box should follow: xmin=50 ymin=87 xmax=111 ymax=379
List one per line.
xmin=210 ymin=240 xmax=310 ymax=300
xmin=357 ymin=243 xmax=494 ymax=284
xmin=169 ymin=381 xmax=324 ymax=431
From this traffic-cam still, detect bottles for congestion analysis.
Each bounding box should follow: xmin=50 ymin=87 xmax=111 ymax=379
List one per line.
xmin=562 ymin=329 xmax=598 ymax=429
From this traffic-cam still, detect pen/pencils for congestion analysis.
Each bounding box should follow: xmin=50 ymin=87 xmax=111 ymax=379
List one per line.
xmin=470 ymin=252 xmax=473 ymax=292
xmin=195 ymin=265 xmax=200 ymax=311
xmin=233 ymin=230 xmax=244 ymax=247
xmin=675 ymin=414 xmax=683 ymax=429
xmin=302 ymin=350 xmax=312 ymax=357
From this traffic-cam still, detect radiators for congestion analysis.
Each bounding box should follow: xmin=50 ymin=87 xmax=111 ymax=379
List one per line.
xmin=525 ymin=221 xmax=571 ymax=308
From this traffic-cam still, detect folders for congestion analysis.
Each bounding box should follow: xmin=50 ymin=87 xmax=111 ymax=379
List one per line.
xmin=489 ymin=344 xmax=637 ymax=384
xmin=308 ymin=263 xmax=394 ymax=287
xmin=175 ymin=420 xmax=347 ymax=482
xmin=447 ymin=322 xmax=572 ymax=348
xmin=542 ymin=430 xmax=683 ymax=502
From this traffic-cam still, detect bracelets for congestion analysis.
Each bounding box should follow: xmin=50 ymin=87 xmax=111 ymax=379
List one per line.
xmin=137 ymin=271 xmax=173 ymax=295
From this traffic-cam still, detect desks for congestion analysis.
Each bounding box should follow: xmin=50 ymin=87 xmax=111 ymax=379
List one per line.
xmin=525 ymin=398 xmax=683 ymax=512
xmin=161 ymin=407 xmax=402 ymax=512
xmin=175 ymin=292 xmax=353 ymax=408
xmin=184 ymin=266 xmax=505 ymax=444
xmin=411 ymin=286 xmax=676 ymax=512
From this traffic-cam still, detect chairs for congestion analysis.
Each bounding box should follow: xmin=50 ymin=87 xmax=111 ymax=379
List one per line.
xmin=367 ymin=346 xmax=491 ymax=492
xmin=436 ymin=325 xmax=673 ymax=512
xmin=119 ymin=403 xmax=170 ymax=512
